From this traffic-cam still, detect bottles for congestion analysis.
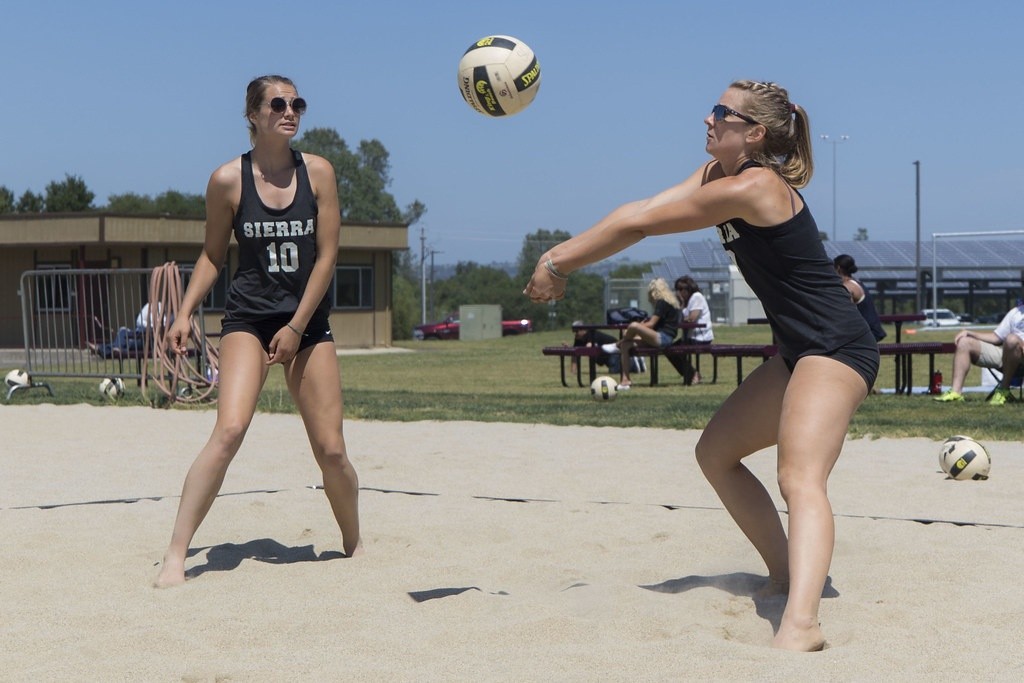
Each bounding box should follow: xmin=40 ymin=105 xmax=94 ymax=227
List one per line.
xmin=933 ymin=368 xmax=943 ymax=395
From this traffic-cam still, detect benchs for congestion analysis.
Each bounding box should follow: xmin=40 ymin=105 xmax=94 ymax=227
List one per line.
xmin=542 ymin=341 xmax=956 ymax=396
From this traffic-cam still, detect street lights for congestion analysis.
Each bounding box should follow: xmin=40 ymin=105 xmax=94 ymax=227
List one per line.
xmin=821 ymin=133 xmax=849 ymax=241
xmin=912 ymin=159 xmax=923 ymax=315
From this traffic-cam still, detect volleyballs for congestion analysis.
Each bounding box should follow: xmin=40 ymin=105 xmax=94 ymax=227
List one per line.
xmin=590 ymin=376 xmax=619 ymax=402
xmin=4 ymin=368 xmax=30 ymax=391
xmin=458 ymin=34 xmax=543 ymax=117
xmin=99 ymin=377 xmax=126 ymax=401
xmin=944 ymin=440 xmax=991 ymax=481
xmin=938 ymin=436 xmax=975 ymax=473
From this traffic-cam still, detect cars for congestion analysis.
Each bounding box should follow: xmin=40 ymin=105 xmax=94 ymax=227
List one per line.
xmin=922 ymin=308 xmax=962 ymax=327
xmin=415 ymin=315 xmax=533 ymax=340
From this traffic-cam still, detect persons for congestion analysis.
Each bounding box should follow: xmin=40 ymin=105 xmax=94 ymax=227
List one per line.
xmin=601 ymin=278 xmax=683 ymax=386
xmin=933 ymin=304 xmax=1024 ymax=405
xmin=156 ymin=75 xmax=359 ymax=588
xmin=833 ymin=255 xmax=887 ymax=343
xmin=524 ymin=79 xmax=880 ymax=654
xmin=85 ymin=301 xmax=174 ymax=358
xmin=569 ymin=320 xmax=646 ymax=374
xmin=665 ymin=275 xmax=714 ymax=385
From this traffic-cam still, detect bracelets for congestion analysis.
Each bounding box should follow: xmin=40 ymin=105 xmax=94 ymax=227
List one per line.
xmin=545 ymin=260 xmax=568 ymax=280
xmin=287 ymin=323 xmax=303 ymax=335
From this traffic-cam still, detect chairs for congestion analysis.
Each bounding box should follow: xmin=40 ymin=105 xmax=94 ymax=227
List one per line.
xmin=985 ymin=365 xmax=1024 ymax=403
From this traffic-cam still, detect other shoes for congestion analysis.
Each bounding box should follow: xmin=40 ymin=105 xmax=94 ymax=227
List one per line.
xmin=688 ymin=371 xmax=702 ymax=385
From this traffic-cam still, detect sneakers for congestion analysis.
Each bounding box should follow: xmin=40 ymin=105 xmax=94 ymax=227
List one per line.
xmin=617 ymin=383 xmax=632 ymax=391
xmin=988 ymin=388 xmax=1010 ymax=405
xmin=934 ymin=391 xmax=965 ymax=403
xmin=602 ymin=343 xmax=620 ymax=353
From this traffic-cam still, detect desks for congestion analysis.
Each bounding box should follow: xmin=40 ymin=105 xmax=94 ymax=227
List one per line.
xmin=571 ymin=323 xmax=706 ymax=384
xmin=749 ymin=312 xmax=926 ymax=394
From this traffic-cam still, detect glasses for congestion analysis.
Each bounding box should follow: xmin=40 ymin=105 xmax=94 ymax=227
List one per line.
xmin=256 ymin=97 xmax=307 ymax=115
xmin=711 ymin=104 xmax=774 ymax=138
